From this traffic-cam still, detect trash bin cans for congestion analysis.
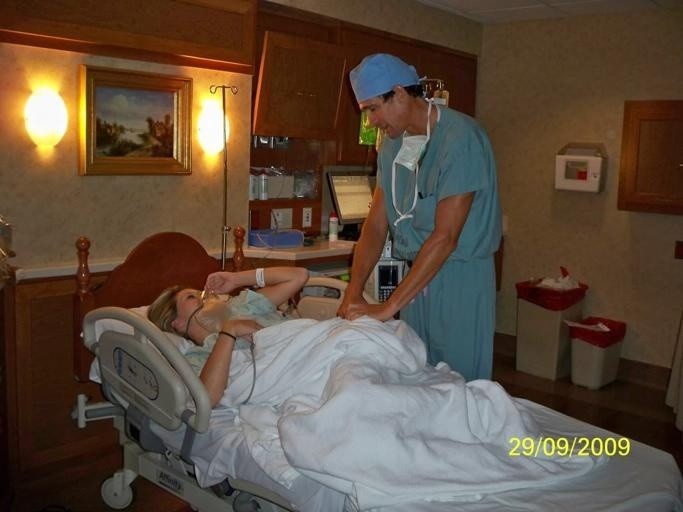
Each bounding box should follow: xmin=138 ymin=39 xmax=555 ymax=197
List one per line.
xmin=563 ymin=315 xmax=628 ymax=391
xmin=515 ymin=264 xmax=589 ymax=381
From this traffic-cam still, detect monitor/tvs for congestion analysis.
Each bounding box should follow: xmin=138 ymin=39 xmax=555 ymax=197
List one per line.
xmin=326 ymin=169 xmax=377 ymax=242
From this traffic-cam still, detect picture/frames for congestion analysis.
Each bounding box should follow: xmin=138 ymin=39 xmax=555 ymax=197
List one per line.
xmin=78 ymin=64 xmax=195 ymax=177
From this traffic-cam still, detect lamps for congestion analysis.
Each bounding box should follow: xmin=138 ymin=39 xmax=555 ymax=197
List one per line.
xmin=73 ymin=224 xmax=662 ymax=512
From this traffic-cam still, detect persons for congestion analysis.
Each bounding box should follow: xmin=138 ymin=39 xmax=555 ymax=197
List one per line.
xmin=147 ymin=267 xmax=309 ymax=407
xmin=146 ymin=116 xmax=159 ymax=157
xmin=161 ymin=114 xmax=173 ymax=156
xmin=336 ymin=53 xmax=503 ymax=381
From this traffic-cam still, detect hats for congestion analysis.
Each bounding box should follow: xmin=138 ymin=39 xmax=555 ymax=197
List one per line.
xmin=348 ymin=53 xmax=418 ymax=102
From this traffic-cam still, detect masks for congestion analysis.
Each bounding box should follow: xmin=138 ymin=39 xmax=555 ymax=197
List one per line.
xmin=391 ymin=98 xmax=441 ymax=227
